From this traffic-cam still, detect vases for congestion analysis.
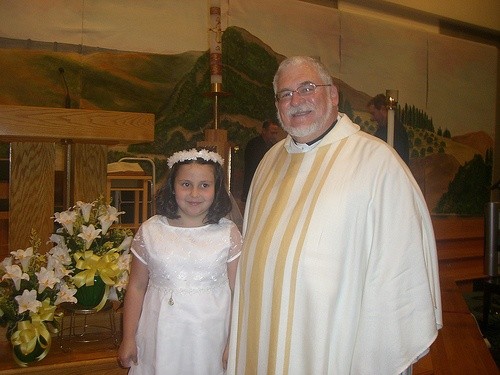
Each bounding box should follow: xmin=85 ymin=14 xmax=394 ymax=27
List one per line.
xmin=10 ymin=319 xmax=52 ymax=368
xmin=70 ymin=264 xmax=109 ymax=308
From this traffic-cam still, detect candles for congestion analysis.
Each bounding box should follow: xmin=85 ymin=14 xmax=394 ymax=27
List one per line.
xmin=386 ymin=99 xmax=395 ymax=148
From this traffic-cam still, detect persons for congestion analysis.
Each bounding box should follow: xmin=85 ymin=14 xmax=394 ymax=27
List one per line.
xmin=226 ymin=55 xmax=443 ymax=375
xmin=367 ymin=94 xmax=409 ymax=165
xmin=241 ymin=119 xmax=280 ymax=203
xmin=118 ymin=147 xmax=244 ymax=375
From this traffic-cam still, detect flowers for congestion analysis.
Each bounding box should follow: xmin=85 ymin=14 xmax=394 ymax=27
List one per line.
xmin=0 ymin=230 xmax=78 ymax=348
xmin=46 ymin=191 xmax=133 ymax=302
xmin=166 ymin=148 xmax=225 ymax=169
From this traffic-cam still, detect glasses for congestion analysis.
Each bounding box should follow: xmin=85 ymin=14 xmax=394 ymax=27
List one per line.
xmin=276 ymin=83 xmax=331 ymax=100
